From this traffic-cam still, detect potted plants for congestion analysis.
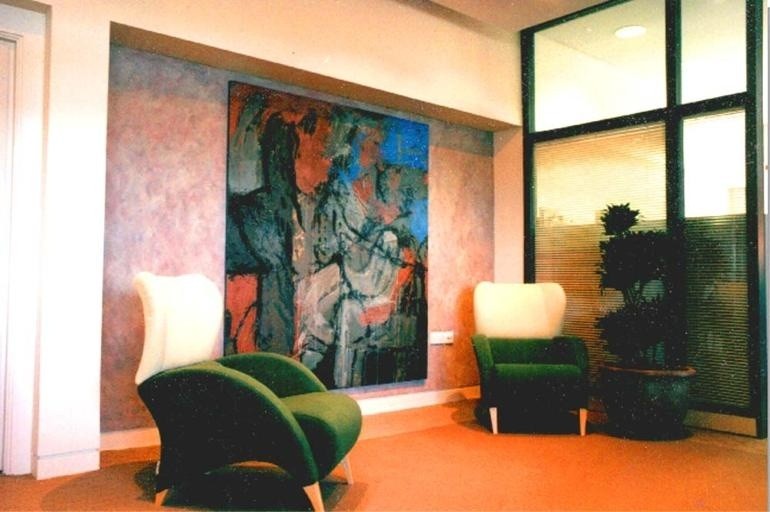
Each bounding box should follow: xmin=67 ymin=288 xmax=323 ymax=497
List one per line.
xmin=594 ymin=204 xmax=697 ymax=439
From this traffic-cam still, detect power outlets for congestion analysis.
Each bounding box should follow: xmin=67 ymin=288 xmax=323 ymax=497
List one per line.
xmin=430 ymin=331 xmax=455 ymax=346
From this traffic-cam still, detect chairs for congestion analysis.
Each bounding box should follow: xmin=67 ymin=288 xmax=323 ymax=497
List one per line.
xmin=134 ymin=271 xmax=363 ymax=512
xmin=471 ymin=279 xmax=591 ymax=435
